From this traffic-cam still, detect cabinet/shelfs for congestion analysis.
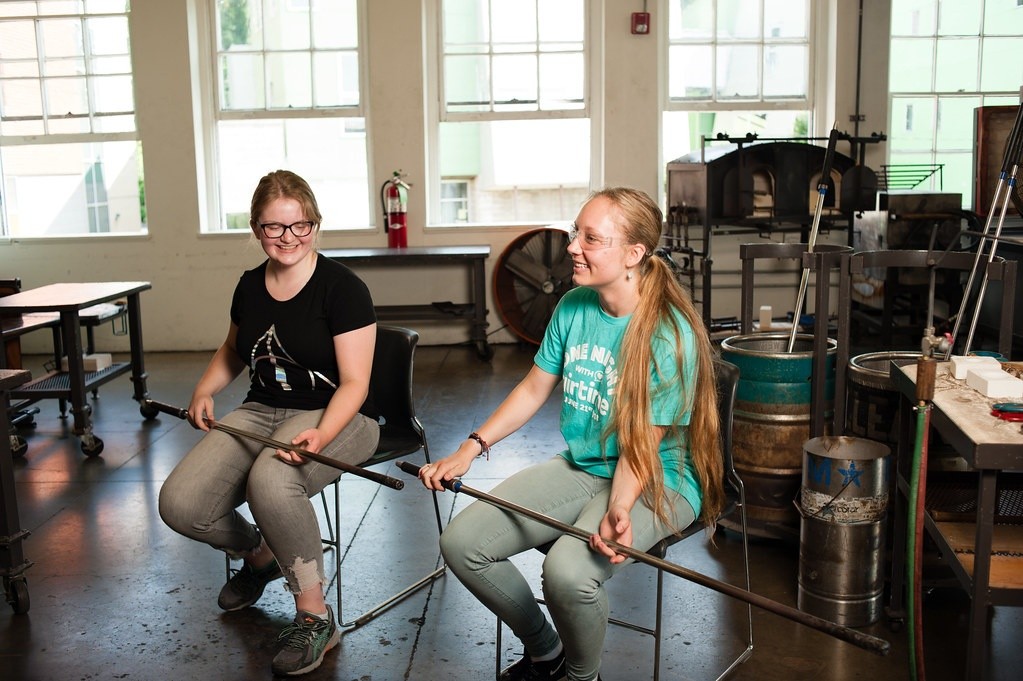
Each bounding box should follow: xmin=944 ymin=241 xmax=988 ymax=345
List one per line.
xmin=0 ymin=368 xmax=33 ymax=615
xmin=317 ymin=243 xmax=492 ymax=362
xmin=886 ymin=358 xmax=1023 ymax=681
xmin=0 ymin=281 xmax=160 ymax=456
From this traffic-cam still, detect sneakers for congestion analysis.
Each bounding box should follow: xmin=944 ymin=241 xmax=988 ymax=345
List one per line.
xmin=500 ymin=647 xmax=568 ymax=681
xmin=568 ymin=672 xmax=602 ymax=681
xmin=273 ymin=604 xmax=339 ymax=675
xmin=218 ymin=557 xmax=284 ymax=611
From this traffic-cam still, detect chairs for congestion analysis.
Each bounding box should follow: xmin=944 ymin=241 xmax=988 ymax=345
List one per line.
xmin=494 ymin=359 xmax=755 ymax=681
xmin=221 ymin=323 xmax=447 ymax=628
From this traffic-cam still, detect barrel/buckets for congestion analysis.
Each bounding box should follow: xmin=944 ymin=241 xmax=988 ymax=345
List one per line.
xmin=848 ymin=351 xmax=941 ymax=437
xmin=968 ymin=350 xmax=1002 ymax=358
xmin=795 ymin=436 xmax=891 ymax=627
xmin=720 ymin=328 xmax=850 ymax=521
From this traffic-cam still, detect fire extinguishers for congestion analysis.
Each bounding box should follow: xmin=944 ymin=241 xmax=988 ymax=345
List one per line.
xmin=379 ymin=169 xmax=414 ymax=249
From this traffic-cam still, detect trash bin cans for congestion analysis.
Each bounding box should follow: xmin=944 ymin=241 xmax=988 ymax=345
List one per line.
xmin=796 ymin=434 xmax=892 ymax=627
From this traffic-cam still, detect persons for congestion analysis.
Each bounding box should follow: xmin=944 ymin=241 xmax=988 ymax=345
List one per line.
xmin=159 ymin=170 xmax=380 ymax=677
xmin=418 ymin=188 xmax=719 ymax=681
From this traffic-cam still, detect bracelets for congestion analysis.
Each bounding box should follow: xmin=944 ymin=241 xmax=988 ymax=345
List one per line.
xmin=468 ymin=432 xmax=489 ymax=462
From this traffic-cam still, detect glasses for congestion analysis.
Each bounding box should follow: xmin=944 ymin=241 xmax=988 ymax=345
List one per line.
xmin=568 ymin=221 xmax=629 ymax=250
xmin=256 ymin=218 xmax=316 ymax=239
